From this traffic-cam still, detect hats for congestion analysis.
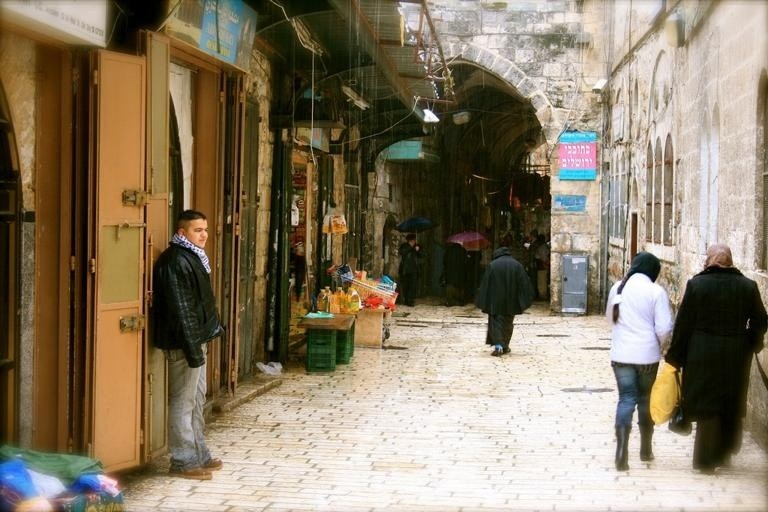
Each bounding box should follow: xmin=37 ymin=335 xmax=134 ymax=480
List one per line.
xmin=406 ymin=233 xmax=416 ymax=240
xmin=493 ymin=246 xmax=512 ymax=258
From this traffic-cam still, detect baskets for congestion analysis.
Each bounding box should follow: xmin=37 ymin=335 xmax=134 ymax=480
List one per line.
xmin=306 ymin=354 xmax=336 ymax=372
xmin=308 ymin=329 xmax=336 ymax=354
xmin=336 ymin=321 xmax=354 ymax=364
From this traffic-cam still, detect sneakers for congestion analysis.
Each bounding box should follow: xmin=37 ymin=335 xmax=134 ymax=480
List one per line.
xmin=204 ymin=457 xmax=222 ymax=470
xmin=504 ymin=348 xmax=511 ymax=353
xmin=492 ymin=345 xmax=503 ymax=356
xmin=169 ymin=467 xmax=212 ymax=480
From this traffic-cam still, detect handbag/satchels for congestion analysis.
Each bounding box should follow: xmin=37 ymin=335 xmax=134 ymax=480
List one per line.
xmin=650 ymin=362 xmax=681 ymax=424
xmin=669 ymin=404 xmax=692 ymax=435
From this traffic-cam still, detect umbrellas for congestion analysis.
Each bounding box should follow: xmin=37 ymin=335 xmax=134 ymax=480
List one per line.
xmin=393 ymin=216 xmax=440 ymax=245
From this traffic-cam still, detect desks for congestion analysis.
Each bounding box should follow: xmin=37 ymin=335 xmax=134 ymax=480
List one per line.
xmin=353 ymin=305 xmax=391 ymax=349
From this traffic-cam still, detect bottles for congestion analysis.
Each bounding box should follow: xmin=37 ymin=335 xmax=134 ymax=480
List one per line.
xmin=317 ymin=286 xmax=361 ymax=315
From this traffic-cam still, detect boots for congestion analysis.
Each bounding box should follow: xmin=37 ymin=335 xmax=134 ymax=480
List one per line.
xmin=616 ymin=425 xmax=630 ymax=470
xmin=639 ymin=424 xmax=655 ymax=461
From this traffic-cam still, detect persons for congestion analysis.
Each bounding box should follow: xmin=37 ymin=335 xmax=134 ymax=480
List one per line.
xmin=664 ymin=243 xmax=768 ymax=475
xmin=152 ymin=210 xmax=226 ymax=479
xmin=398 ymin=230 xmax=546 ymax=356
xmin=606 ymin=252 xmax=674 ymax=471
